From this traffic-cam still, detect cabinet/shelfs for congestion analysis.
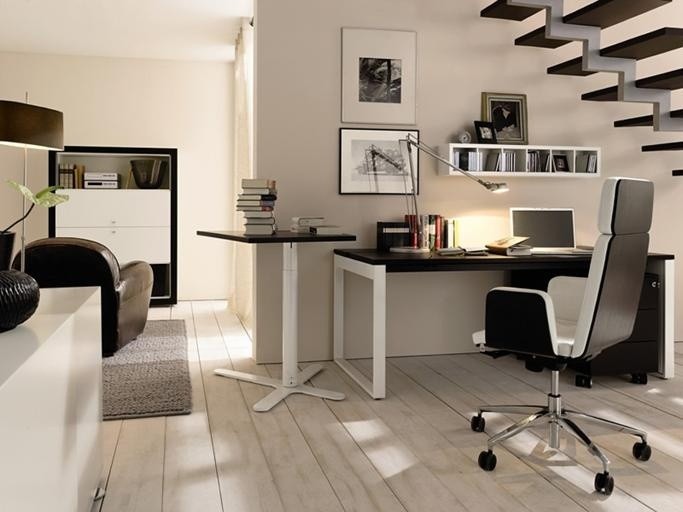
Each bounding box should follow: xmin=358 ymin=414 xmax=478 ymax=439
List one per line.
xmin=575 ymin=277 xmax=659 ymax=387
xmin=49 ymin=146 xmax=177 ymax=307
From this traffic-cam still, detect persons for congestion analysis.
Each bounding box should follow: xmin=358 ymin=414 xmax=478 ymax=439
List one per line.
xmin=494 ymin=101 xmax=513 ymax=130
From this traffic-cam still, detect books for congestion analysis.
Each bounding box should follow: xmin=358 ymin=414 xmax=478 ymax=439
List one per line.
xmin=451 ymin=150 xmax=596 ymax=173
xmin=234 ymin=178 xmax=279 ymax=236
xmin=147 ymin=159 xmax=162 ymax=183
xmin=288 ymin=224 xmax=308 ymax=233
xmin=407 ymin=213 xmax=460 ymax=250
xmin=309 ymin=226 xmax=341 ymax=235
xmin=462 ymin=244 xmax=489 ymax=256
xmin=291 ymin=217 xmax=327 ymax=226
xmin=438 ymin=247 xmax=464 ymax=256
xmin=58 ymin=164 xmax=85 ymax=189
xmin=485 ymin=235 xmax=533 ymax=255
xmin=124 ymin=166 xmax=133 ymax=189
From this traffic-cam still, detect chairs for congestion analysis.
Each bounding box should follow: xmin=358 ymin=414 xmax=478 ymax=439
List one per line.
xmin=11 ymin=237 xmax=155 ymax=358
xmin=470 ymin=177 xmax=654 ymax=496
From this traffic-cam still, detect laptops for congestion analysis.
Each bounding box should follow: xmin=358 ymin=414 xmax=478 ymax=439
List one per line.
xmin=510 ymin=207 xmax=576 ymax=255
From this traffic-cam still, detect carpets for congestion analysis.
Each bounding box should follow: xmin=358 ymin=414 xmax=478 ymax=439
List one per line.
xmin=103 ymin=319 xmax=192 ymax=421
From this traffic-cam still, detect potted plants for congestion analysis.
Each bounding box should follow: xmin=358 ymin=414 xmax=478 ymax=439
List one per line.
xmin=0 ymin=179 xmax=70 ymax=269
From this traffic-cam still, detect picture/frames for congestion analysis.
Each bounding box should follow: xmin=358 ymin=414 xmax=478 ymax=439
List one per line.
xmin=473 ymin=92 xmax=527 ymax=144
xmin=339 ymin=27 xmax=420 ymax=196
xmin=553 ymin=155 xmax=568 ymax=171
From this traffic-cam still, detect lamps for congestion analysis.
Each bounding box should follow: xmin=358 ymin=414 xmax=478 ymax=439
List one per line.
xmin=1 ymin=92 xmax=64 ymax=273
xmin=389 ymin=133 xmax=508 ymax=252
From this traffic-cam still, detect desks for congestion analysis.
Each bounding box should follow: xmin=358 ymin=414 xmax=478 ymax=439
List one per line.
xmin=197 ymin=230 xmax=356 ymax=413
xmin=334 ymin=246 xmax=675 ymax=400
